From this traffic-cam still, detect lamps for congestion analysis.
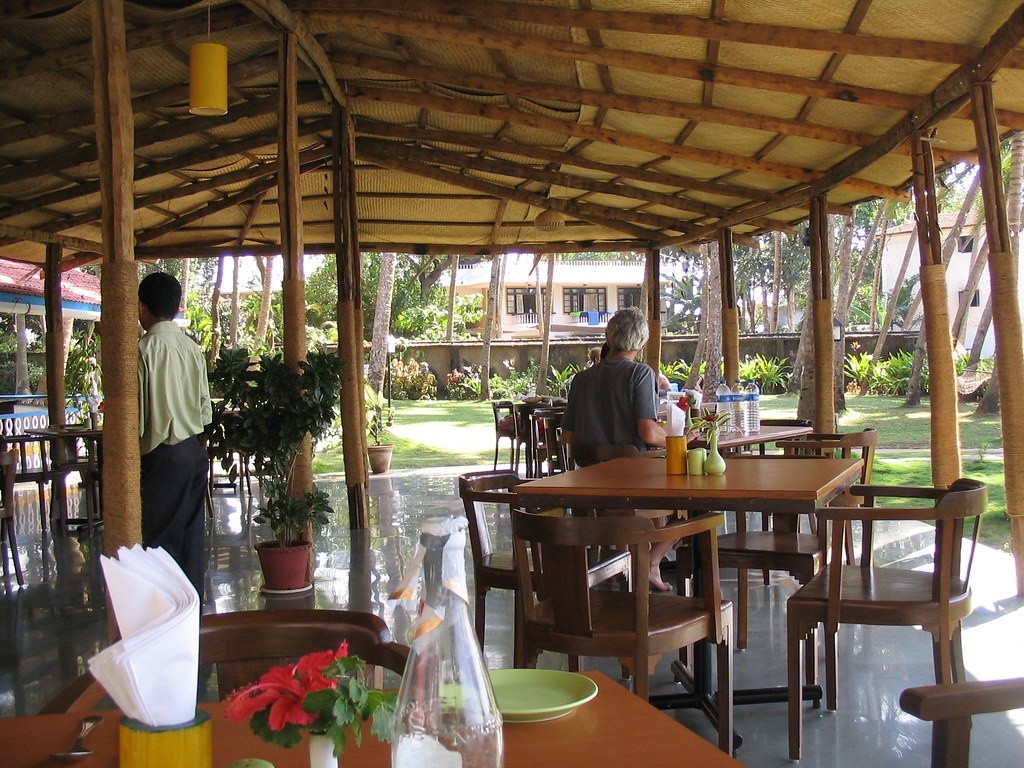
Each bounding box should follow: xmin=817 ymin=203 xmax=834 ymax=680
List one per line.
xmin=534 ymin=206 xmax=566 ymax=231
xmin=188 ymin=0 xmax=228 ymax=116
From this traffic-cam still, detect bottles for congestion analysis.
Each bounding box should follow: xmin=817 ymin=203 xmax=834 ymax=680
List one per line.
xmin=687 ymin=447 xmax=707 ymax=475
xmin=390 ymin=517 xmax=504 ymax=768
xmin=729 ymin=379 xmax=744 ymax=432
xmin=715 ymin=380 xmax=731 ymax=435
xmin=744 ymin=379 xmax=760 ymax=432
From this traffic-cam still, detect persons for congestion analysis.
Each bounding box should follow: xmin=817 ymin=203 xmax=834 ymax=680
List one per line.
xmin=659 ymin=372 xmax=669 ymax=395
xmin=561 ymin=306 xmax=685 ymax=591
xmin=136 ymin=273 xmax=214 ymax=696
xmin=589 ymin=347 xmax=603 ymax=368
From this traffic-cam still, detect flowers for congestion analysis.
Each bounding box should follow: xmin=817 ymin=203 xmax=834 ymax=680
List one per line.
xmin=676 ymin=394 xmax=697 ymax=412
xmin=214 ymin=635 xmax=399 ymax=760
xmin=687 ymin=407 xmax=743 ymax=445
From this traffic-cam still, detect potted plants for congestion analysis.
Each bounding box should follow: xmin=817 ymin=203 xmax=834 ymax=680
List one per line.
xmin=364 ymin=383 xmax=394 ymax=474
xmin=205 ymin=345 xmax=345 ymax=596
xmin=69 ymin=335 xmax=104 ymax=490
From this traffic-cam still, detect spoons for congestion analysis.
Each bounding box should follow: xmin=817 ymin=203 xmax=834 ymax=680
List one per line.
xmin=47 ymin=714 xmax=104 ymax=759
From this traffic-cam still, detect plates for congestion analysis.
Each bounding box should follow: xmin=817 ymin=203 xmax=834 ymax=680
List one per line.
xmin=440 ymin=667 xmax=598 ymax=722
xmin=66 ymin=427 xmax=90 ymax=432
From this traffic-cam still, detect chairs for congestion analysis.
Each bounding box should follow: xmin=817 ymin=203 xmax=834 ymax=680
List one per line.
xmin=0 ymin=431 xmax=105 ymax=587
xmin=194 ymin=606 xmax=434 ymax=704
xmin=457 ymin=400 xmax=1024 ymax=768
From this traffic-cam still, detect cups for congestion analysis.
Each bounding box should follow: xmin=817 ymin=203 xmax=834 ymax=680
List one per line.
xmin=84 ymin=418 xmax=92 ymax=431
xmin=665 ymin=436 xmax=687 ymax=474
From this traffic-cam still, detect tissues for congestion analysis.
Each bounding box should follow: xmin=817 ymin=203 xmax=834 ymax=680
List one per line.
xmin=687 ymin=389 xmax=704 ymax=409
xmin=87 ymin=542 xmax=201 ymax=728
xmin=665 ymin=402 xmax=686 ymax=437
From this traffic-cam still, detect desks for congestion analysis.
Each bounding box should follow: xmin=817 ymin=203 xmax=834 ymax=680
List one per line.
xmin=656 ymin=419 xmax=814 ymax=579
xmin=513 ymin=459 xmax=865 ymax=757
xmin=0 ymin=669 xmax=752 ymax=768
xmin=24 ymin=426 xmax=104 ymax=535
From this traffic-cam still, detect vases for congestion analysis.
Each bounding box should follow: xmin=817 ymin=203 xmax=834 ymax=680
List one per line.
xmin=685 ymin=407 xmax=693 ymax=428
xmin=703 ymin=430 xmax=727 ymax=475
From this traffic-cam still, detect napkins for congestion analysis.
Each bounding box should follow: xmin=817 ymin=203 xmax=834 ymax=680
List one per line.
xmin=86 ymin=540 xmax=202 ymax=729
